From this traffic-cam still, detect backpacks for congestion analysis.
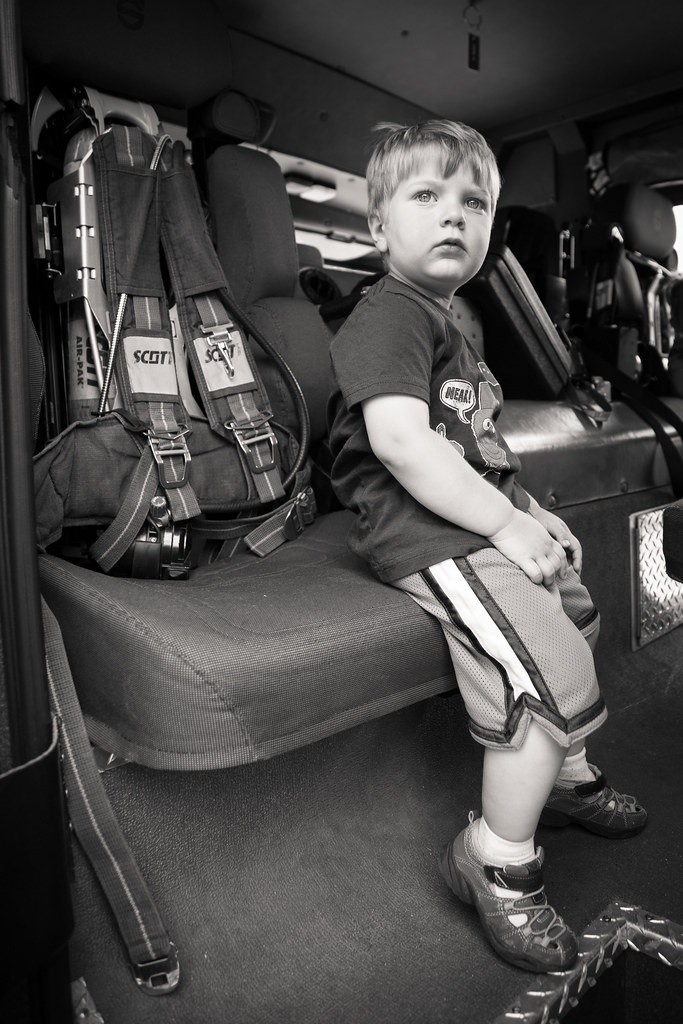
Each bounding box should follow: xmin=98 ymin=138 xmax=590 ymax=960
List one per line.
xmin=545 ymin=215 xmax=683 ymax=500
xmin=31 ymin=84 xmax=318 ymax=581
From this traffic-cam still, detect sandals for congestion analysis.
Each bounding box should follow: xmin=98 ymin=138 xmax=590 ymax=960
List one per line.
xmin=539 ymin=763 xmax=648 ymax=839
xmin=439 ymin=810 xmax=579 ymax=972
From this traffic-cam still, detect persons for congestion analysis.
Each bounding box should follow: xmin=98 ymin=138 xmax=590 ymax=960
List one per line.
xmin=329 ymin=118 xmax=649 ymax=971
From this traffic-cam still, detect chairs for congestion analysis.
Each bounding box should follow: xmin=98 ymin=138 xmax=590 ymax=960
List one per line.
xmin=19 ymin=500 xmax=459 ymax=1001
